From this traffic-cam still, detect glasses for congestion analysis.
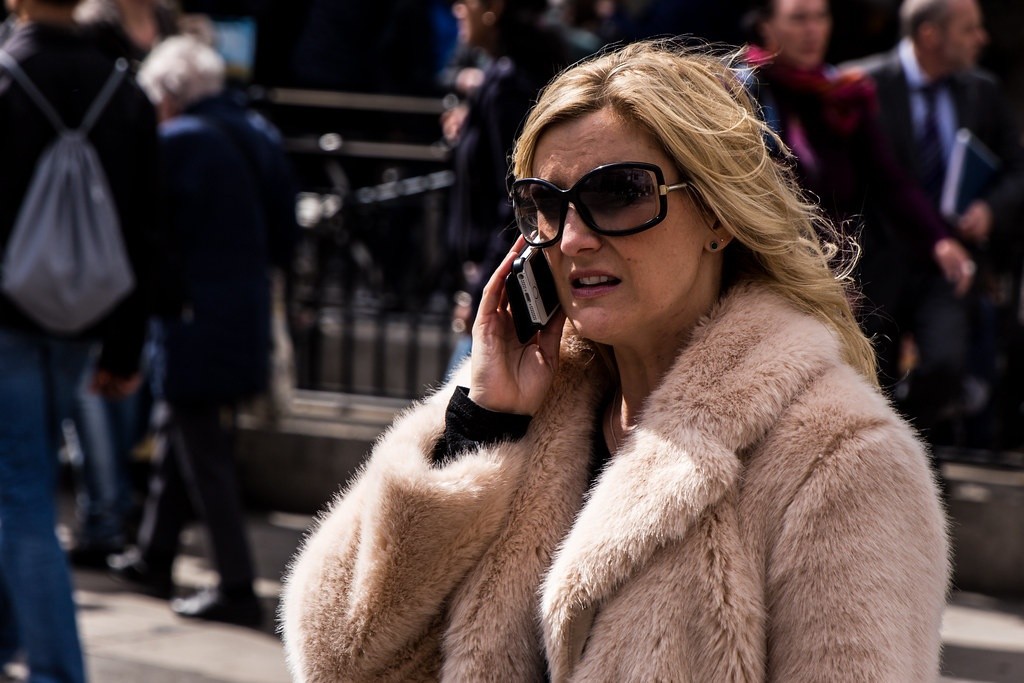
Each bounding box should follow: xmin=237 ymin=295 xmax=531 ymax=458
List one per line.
xmin=511 ymin=162 xmax=693 ymax=247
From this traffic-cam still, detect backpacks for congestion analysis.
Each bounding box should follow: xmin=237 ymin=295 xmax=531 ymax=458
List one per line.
xmin=0 ymin=50 xmax=136 ymax=333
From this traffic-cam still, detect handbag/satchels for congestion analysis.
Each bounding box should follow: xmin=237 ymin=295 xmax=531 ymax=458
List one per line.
xmin=231 ymin=279 xmax=299 ymax=430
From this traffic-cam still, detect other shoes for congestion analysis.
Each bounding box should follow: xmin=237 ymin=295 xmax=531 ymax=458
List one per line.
xmin=170 ymin=585 xmax=252 ymax=625
xmin=59 ymin=543 xmax=125 ymax=573
xmin=107 ymin=561 xmax=175 ymax=599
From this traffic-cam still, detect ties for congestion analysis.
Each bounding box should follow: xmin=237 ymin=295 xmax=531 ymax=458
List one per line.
xmin=918 ymin=85 xmax=945 ymax=211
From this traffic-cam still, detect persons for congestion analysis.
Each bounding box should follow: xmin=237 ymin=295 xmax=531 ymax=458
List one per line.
xmin=442 ymin=0 xmax=646 ymax=335
xmin=278 ymin=41 xmax=950 ymax=683
xmin=0 ymin=0 xmax=285 ymax=683
xmin=739 ymin=0 xmax=1024 ymax=466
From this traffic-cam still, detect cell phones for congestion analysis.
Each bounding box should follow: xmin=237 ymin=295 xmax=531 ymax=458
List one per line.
xmin=514 ymin=235 xmax=560 ymax=327
